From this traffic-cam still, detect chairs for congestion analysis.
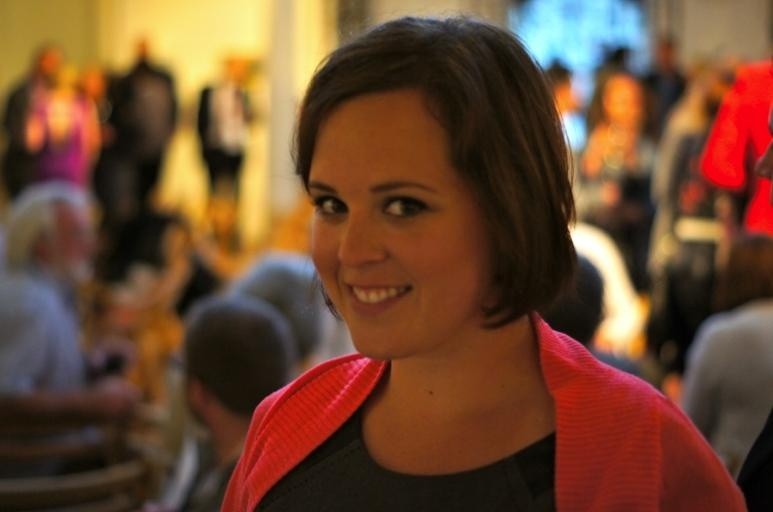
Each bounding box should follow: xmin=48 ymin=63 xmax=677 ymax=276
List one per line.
xmin=0 ymin=378 xmax=155 ymax=512
